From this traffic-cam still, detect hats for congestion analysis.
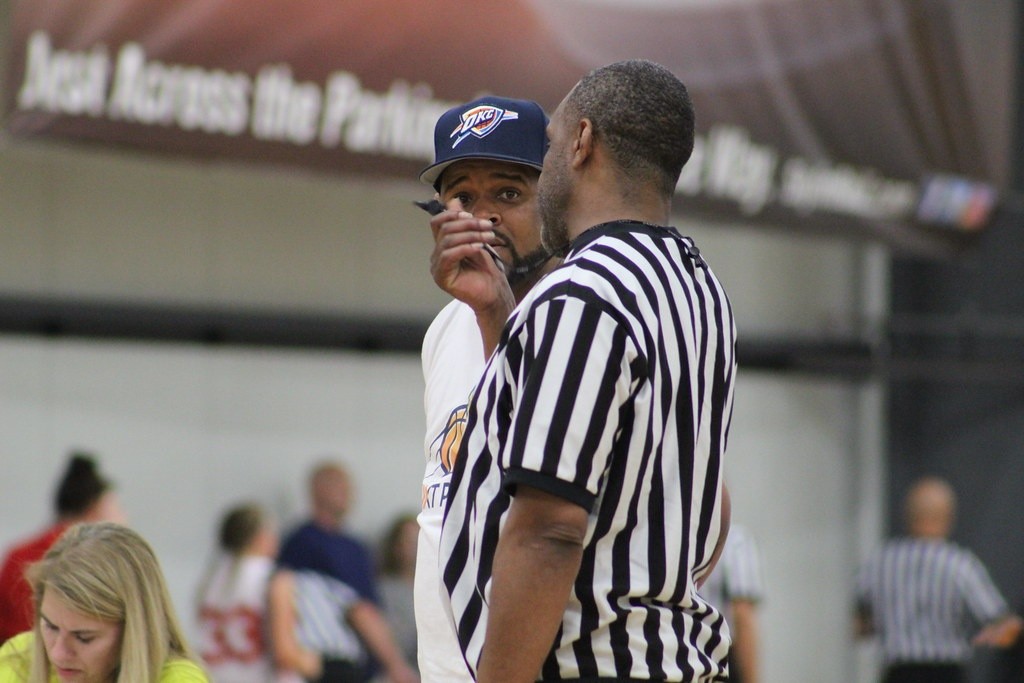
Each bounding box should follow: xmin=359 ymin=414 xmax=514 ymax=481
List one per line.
xmin=418 ymin=94 xmax=550 ymax=186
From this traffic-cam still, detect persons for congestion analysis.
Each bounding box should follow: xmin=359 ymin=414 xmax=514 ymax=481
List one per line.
xmin=427 ymin=59 xmax=739 ymax=683
xmin=195 ymin=466 xmax=421 ymax=683
xmin=696 ymin=526 xmax=777 ymax=683
xmin=0 ymin=523 xmax=207 ymax=683
xmin=414 ymin=96 xmax=733 ymax=683
xmin=847 ymin=476 xmax=1024 ymax=683
xmin=0 ymin=453 xmax=124 ymax=648
